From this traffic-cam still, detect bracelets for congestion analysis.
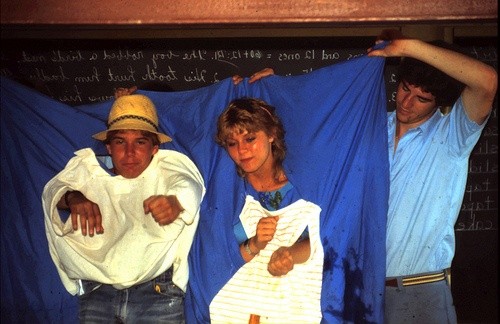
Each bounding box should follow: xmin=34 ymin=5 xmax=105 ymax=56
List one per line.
xmin=244 ymin=238 xmax=255 ymax=258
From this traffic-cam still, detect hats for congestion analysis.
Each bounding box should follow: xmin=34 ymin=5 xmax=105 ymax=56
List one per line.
xmin=90 ymin=94 xmax=175 ymax=144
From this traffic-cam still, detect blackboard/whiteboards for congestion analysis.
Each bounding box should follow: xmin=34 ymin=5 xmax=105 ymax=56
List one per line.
xmin=0 ymin=32 xmax=500 ymax=237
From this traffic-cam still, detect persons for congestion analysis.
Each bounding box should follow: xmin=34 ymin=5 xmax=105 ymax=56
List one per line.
xmin=42 ymin=37 xmax=497 ymax=324
